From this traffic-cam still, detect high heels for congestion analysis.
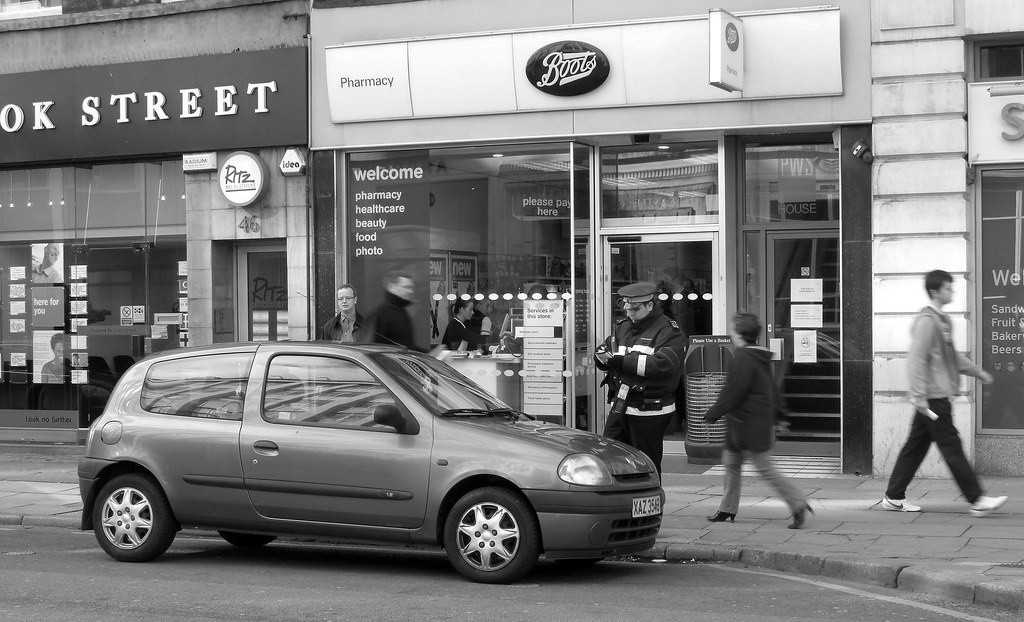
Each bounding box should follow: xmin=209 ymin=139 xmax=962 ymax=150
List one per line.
xmin=706 ymin=510 xmax=736 ymax=523
xmin=788 ymin=505 xmax=814 ymax=529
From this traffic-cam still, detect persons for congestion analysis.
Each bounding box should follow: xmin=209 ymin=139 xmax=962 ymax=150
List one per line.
xmin=42 ymin=334 xmax=66 ymax=384
xmin=704 ymin=313 xmax=815 ymax=530
xmin=442 ymin=298 xmax=474 ymax=351
xmin=883 ymin=270 xmax=1008 ymax=518
xmin=595 ymin=283 xmax=688 ymax=486
xmin=32 ymin=242 xmax=64 ymax=286
xmin=72 ymin=354 xmax=80 ymax=365
xmin=319 ymin=285 xmax=365 ymax=342
xmin=368 ymin=271 xmax=416 ymax=351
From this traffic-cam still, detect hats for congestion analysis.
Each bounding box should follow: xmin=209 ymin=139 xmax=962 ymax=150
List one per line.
xmin=618 ymin=282 xmax=657 ymax=309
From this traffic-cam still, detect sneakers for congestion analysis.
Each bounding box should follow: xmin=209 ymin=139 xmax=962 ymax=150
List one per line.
xmin=883 ymin=494 xmax=921 ymax=511
xmin=969 ymin=496 xmax=1008 ymax=518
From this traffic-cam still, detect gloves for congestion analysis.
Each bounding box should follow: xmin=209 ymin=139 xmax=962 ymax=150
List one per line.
xmin=595 ymin=345 xmax=623 ymax=369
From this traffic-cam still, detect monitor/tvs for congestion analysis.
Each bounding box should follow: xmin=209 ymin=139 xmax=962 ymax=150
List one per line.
xmin=500 ymin=313 xmax=513 ymax=336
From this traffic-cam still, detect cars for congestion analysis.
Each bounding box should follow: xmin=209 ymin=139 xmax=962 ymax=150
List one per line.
xmin=772 ymin=324 xmax=840 ymax=435
xmin=77 ymin=339 xmax=665 ymax=582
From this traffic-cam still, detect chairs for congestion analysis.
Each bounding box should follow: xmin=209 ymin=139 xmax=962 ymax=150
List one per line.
xmin=82 ymin=356 xmax=112 ymax=379
xmin=114 ymin=355 xmax=136 ymax=374
xmin=316 ymin=393 xmax=344 ymax=425
xmin=270 ymin=376 xmax=314 ymax=422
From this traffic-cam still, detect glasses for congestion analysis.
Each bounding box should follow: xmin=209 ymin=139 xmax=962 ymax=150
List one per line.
xmin=338 ymin=296 xmax=355 ymax=300
